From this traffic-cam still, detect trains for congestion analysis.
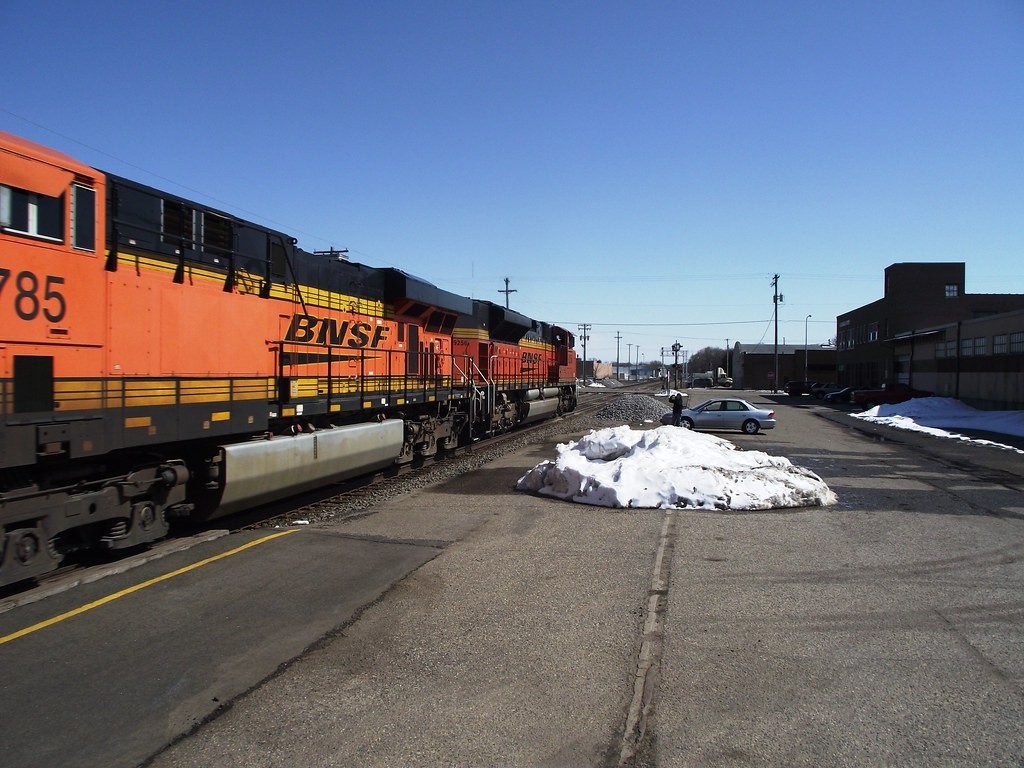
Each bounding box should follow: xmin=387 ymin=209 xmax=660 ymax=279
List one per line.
xmin=0 ymin=129 xmax=580 ymax=601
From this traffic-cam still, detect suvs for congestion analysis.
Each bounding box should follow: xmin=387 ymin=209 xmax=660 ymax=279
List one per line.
xmin=810 ymin=381 xmax=847 ymax=400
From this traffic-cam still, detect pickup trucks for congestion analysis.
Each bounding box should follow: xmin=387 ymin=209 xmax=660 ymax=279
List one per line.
xmin=849 ymin=382 xmax=936 ymax=411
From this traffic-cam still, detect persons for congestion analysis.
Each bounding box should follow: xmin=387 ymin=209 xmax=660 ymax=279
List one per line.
xmin=668 ymin=393 xmax=683 ymax=427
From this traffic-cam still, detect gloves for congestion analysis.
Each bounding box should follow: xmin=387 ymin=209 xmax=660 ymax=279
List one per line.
xmin=670 ymin=396 xmax=672 ymax=400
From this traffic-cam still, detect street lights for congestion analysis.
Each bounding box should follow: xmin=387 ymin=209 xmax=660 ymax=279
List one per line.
xmin=805 ymin=314 xmax=814 ymax=388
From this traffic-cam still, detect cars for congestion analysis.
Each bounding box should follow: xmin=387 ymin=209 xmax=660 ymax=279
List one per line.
xmin=659 ymin=398 xmax=776 ymax=436
xmin=823 ymin=387 xmax=854 ymax=404
